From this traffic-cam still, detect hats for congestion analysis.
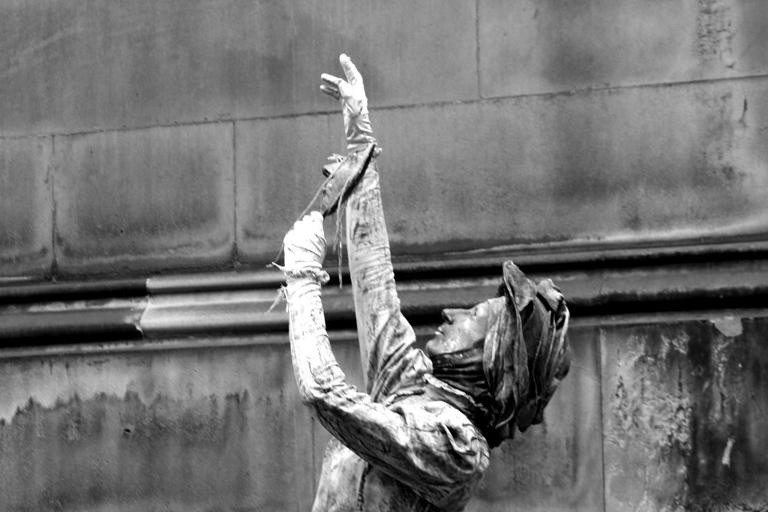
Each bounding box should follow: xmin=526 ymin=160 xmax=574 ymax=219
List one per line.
xmin=501 ymin=260 xmax=572 ymax=433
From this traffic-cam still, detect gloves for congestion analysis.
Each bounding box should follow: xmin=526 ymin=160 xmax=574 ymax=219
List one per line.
xmin=271 ymin=211 xmax=331 ymax=286
xmin=318 ymin=52 xmax=383 ymax=159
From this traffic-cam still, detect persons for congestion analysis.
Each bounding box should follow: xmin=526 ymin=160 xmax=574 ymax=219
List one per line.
xmin=283 ymin=52 xmax=570 ymax=512
